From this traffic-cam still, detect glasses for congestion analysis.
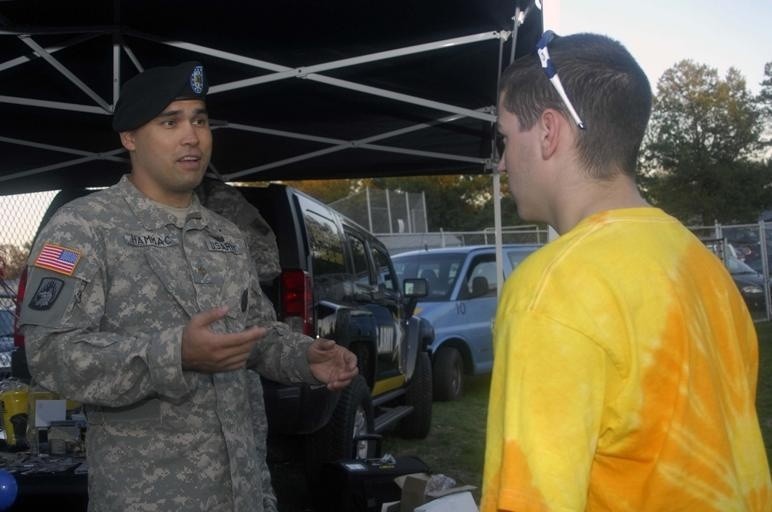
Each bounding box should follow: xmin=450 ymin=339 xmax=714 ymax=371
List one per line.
xmin=535 ymin=27 xmax=585 ymax=129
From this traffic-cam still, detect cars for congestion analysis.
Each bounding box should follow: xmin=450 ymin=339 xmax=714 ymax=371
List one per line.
xmin=696 ymin=208 xmax=772 ymax=314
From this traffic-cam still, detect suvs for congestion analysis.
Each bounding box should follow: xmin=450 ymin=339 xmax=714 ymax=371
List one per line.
xmin=390 ymin=240 xmax=548 ymax=403
xmin=8 ymin=182 xmax=441 ymax=475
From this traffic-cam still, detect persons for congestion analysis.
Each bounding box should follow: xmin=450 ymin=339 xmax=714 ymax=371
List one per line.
xmin=11 ymin=59 xmax=361 ymax=512
xmin=474 ymin=30 xmax=772 ymax=512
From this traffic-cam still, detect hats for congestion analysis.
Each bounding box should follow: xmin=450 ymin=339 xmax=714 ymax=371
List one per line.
xmin=108 ymin=59 xmax=211 ymax=133
